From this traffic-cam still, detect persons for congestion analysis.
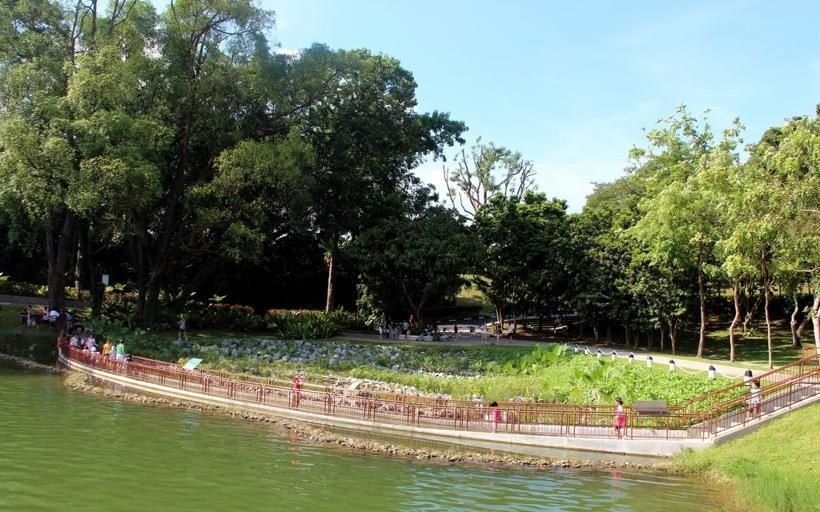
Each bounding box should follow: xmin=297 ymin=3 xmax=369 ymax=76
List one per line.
xmin=377 ymin=314 xmax=567 ymax=344
xmin=742 ymin=311 xmax=753 ymax=336
xmin=292 ymin=375 xmax=303 ymax=409
xmin=489 ymin=400 xmax=500 ymax=433
xmin=176 ymin=315 xmax=189 ymax=341
xmin=17 ymin=303 xmax=133 ymax=374
xmin=745 ymin=381 xmax=762 ymax=422
xmin=611 ymin=396 xmax=626 ymax=439
